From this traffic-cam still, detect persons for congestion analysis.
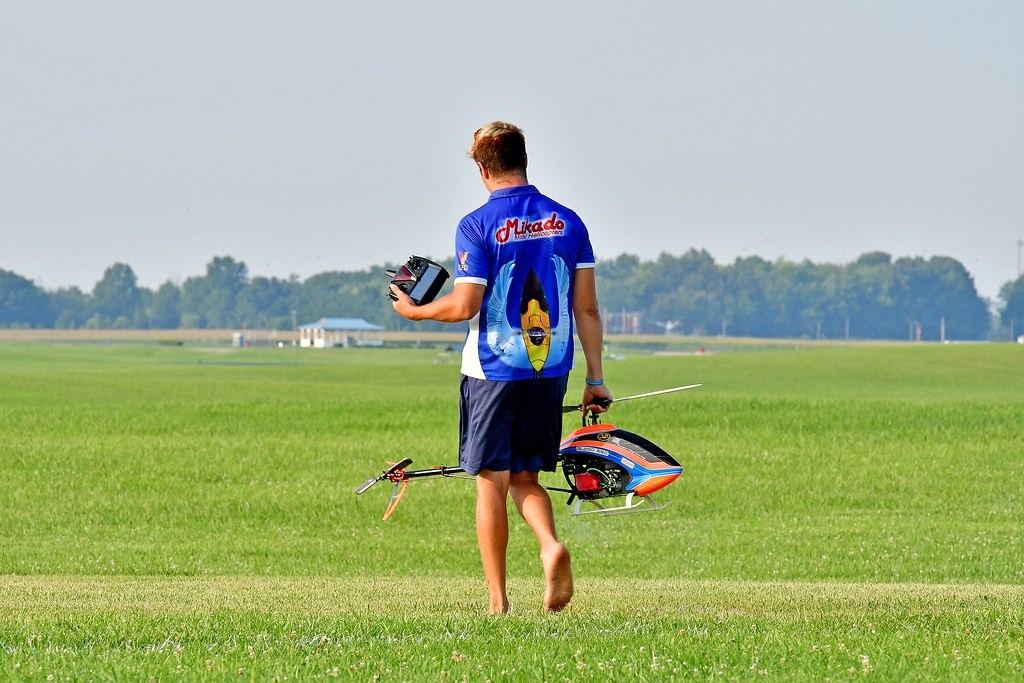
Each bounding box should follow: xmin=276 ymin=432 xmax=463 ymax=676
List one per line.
xmin=391 ymin=120 xmax=614 ymax=618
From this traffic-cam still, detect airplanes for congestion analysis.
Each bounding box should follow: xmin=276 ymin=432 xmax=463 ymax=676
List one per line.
xmin=353 ymin=382 xmax=705 ymax=516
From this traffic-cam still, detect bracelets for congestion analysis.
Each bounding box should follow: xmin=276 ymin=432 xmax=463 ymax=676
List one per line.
xmin=585 ymin=378 xmax=604 ymax=386
